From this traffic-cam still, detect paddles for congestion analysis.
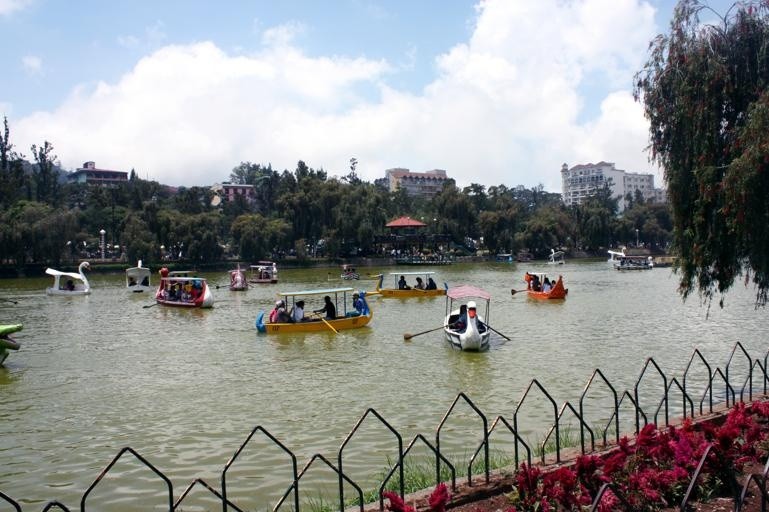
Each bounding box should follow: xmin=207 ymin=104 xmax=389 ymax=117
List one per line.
xmin=405 ymin=324 xmax=450 ymax=340
xmin=0 ymin=297 xmax=19 ymax=305
xmin=143 ymin=299 xmax=165 ymax=308
xmin=512 ymin=289 xmax=528 ymax=296
xmin=216 ymin=285 xmax=232 ymax=289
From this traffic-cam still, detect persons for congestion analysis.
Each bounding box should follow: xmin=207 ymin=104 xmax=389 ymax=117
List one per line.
xmin=269 ymin=299 xmax=312 ymax=323
xmin=164 ymin=273 xmax=202 ymax=303
xmin=62 ymin=280 xmax=75 ymax=290
xmin=414 ymin=276 xmax=426 ymax=290
xmin=399 ymin=275 xmax=411 ymax=290
xmin=530 ymin=275 xmax=556 ymax=293
xmin=346 ymin=292 xmax=365 ymax=318
xmin=129 ymin=277 xmax=137 ymax=287
xmin=425 ymin=277 xmax=436 ymax=290
xmin=344 ymin=268 xmax=356 ymax=275
xmin=313 ymin=295 xmax=335 ymax=320
xmin=142 ymin=277 xmax=149 ymax=286
xmin=452 ymin=311 xmax=479 ymax=334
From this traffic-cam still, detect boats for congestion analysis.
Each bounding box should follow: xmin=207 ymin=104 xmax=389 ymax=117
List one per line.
xmin=604 ymin=246 xmax=682 ymax=273
xmin=493 ymin=248 xmax=566 ymax=266
xmin=124 ymin=258 xmax=278 ymax=310
xmin=444 ymin=285 xmax=492 ymax=352
xmin=525 ymin=271 xmax=567 ymax=299
xmin=44 ymin=262 xmax=92 ymax=295
xmin=340 ymin=272 xmax=360 ymax=280
xmin=256 ymin=287 xmax=381 ymax=333
xmin=373 ymin=272 xmax=448 ymax=298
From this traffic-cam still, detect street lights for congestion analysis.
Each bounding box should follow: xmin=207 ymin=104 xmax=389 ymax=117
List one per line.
xmin=99 ymin=229 xmax=106 ymax=262
xmin=635 ymin=229 xmax=640 ymax=247
